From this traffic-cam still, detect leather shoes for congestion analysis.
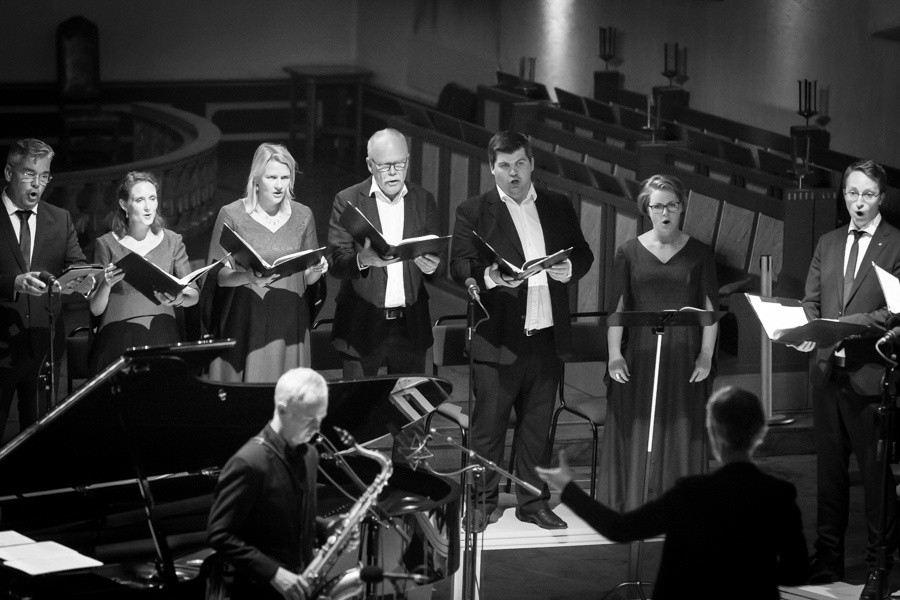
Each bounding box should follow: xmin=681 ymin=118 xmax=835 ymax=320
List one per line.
xmin=516 ymin=508 xmax=567 ymax=529
xmin=463 ymin=507 xmax=489 ymax=532
xmin=860 ymin=568 xmax=896 ymax=600
xmin=810 ymin=560 xmax=844 ymax=583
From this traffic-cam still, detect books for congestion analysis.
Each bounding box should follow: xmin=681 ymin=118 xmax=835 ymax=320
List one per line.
xmin=744 ymin=293 xmax=871 ymax=346
xmin=220 ymin=223 xmax=327 ymax=283
xmin=113 ymin=252 xmax=232 ymax=305
xmin=338 ymin=201 xmax=452 ymax=260
xmin=471 ymin=231 xmax=574 ymax=281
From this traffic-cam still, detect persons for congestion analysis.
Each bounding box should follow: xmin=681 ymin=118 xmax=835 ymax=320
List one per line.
xmin=0 ymin=137 xmax=92 ymax=441
xmin=209 ymin=367 xmax=359 ymax=600
xmin=450 ymin=129 xmax=596 ymax=527
xmin=90 ymin=171 xmax=199 ymax=375
xmin=200 ymin=143 xmax=330 ymax=385
xmin=608 ymin=174 xmax=718 ymax=516
xmin=786 ymin=160 xmax=900 ymax=600
xmin=328 ymin=128 xmax=445 ymax=379
xmin=534 ymin=387 xmax=810 ymax=600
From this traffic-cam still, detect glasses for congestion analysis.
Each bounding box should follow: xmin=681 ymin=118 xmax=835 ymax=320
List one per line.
xmin=844 ymin=188 xmax=882 ymax=199
xmin=8 ymin=163 xmax=53 ymax=184
xmin=648 ymin=199 xmax=681 ymax=214
xmin=369 ymin=156 xmax=408 ymax=172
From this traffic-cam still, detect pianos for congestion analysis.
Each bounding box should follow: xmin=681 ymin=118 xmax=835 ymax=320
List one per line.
xmin=0 ymin=342 xmax=460 ymax=600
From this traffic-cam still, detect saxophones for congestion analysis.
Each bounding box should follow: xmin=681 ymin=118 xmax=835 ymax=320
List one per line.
xmin=300 ymin=427 xmax=394 ymax=600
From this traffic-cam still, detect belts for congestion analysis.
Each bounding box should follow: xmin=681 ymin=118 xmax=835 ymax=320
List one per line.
xmin=344 ymin=288 xmax=428 ymax=319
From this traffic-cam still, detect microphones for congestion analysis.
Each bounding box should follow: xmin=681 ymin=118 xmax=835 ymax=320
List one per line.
xmin=878 ymin=327 xmax=900 ymax=344
xmin=465 ymin=277 xmax=480 ymax=301
xmin=39 ymin=272 xmax=60 ymax=287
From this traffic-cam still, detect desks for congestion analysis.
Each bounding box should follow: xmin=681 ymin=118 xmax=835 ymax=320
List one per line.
xmin=283 ymin=63 xmax=373 ymax=178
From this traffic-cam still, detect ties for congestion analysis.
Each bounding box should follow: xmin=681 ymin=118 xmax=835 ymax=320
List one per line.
xmin=843 ymin=230 xmax=868 ymax=307
xmin=15 ymin=210 xmax=33 ymax=273
